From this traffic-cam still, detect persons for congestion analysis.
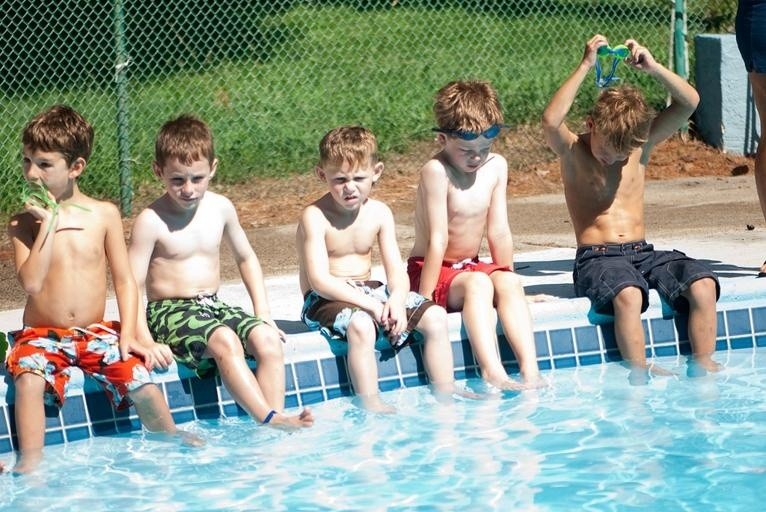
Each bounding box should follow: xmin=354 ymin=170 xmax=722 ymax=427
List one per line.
xmin=128 ymin=119 xmax=313 ymax=436
xmin=543 ymin=35 xmax=724 ymax=382
xmin=735 ymin=0 xmax=765 ymax=276
xmin=294 ymin=125 xmax=483 ymax=415
xmin=6 ymin=104 xmax=203 ymax=477
xmin=408 ymin=81 xmax=548 ymax=395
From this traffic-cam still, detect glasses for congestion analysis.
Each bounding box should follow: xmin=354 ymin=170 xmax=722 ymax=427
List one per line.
xmin=594 ymin=43 xmax=632 ymax=89
xmin=430 ymin=122 xmax=501 ymax=143
